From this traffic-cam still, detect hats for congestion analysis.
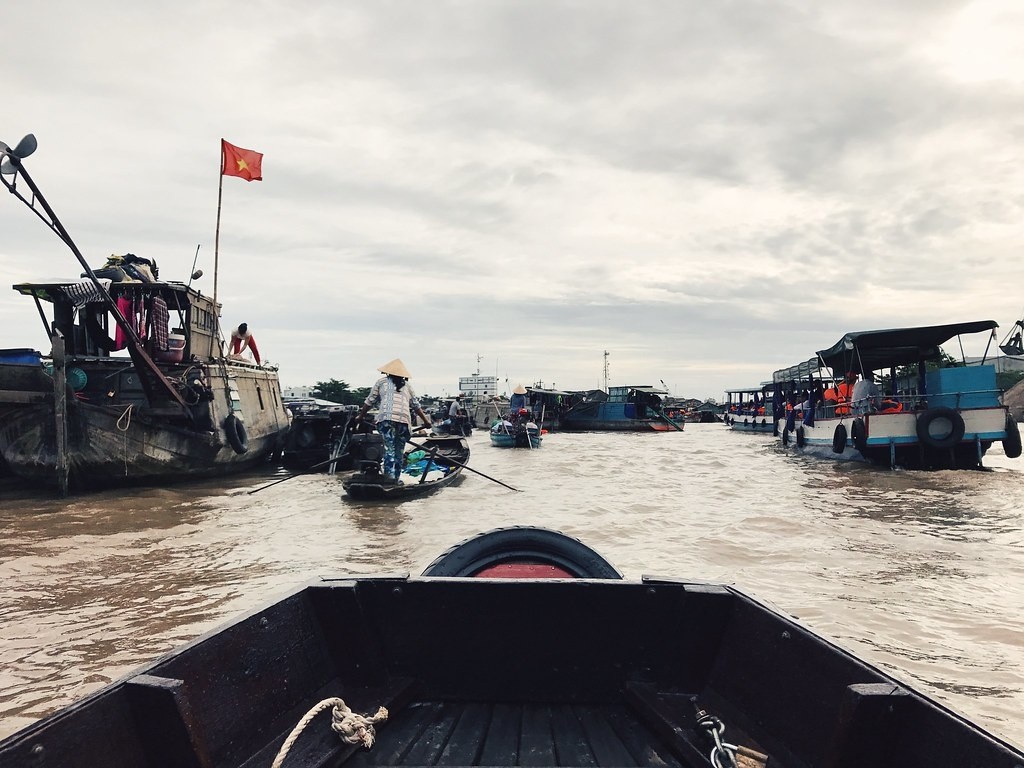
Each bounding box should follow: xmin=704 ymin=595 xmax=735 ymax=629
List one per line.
xmin=512 ymin=385 xmax=527 ymax=394
xmin=377 ymin=359 xmax=411 ymax=378
xmin=846 ymin=372 xmax=858 ymax=379
xmin=862 ymin=369 xmax=877 ymax=376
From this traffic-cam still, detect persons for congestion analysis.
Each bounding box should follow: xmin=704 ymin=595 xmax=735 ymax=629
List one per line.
xmin=730 ymin=397 xmax=765 ymax=415
xmin=785 ymin=369 xmax=881 ymax=419
xmin=225 ymin=322 xmax=260 ymax=366
xmin=448 ymin=397 xmax=464 ymax=437
xmin=355 ymin=358 xmax=432 ymax=486
xmin=509 ymin=384 xmax=528 ymax=423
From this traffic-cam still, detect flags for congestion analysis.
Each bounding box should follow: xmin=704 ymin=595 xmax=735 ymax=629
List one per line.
xmin=222 ymin=140 xmax=263 ymax=181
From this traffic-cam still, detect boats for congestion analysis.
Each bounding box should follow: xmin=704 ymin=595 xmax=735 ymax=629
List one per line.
xmin=341 ymin=426 xmax=470 ymax=501
xmin=773 ymin=319 xmax=1010 ymax=471
xmin=721 ymin=381 xmax=775 ymax=432
xmin=277 ymin=379 xmax=725 ymax=469
xmin=0 ymin=134 xmax=291 ymax=495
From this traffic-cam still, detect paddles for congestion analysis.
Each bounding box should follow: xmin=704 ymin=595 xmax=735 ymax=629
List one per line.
xmin=358 ymin=418 xmax=517 ymax=491
xmin=248 ymin=425 xmax=426 ymax=494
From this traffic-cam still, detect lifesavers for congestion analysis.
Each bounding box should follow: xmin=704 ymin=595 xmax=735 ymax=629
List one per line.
xmin=851 ymin=418 xmax=866 ymax=449
xmin=224 ymin=415 xmax=249 ymax=455
xmin=916 ymin=406 xmax=965 ymax=449
xmin=1002 ymin=411 xmax=1023 ymax=458
xmin=832 ymin=424 xmax=847 ymax=454
